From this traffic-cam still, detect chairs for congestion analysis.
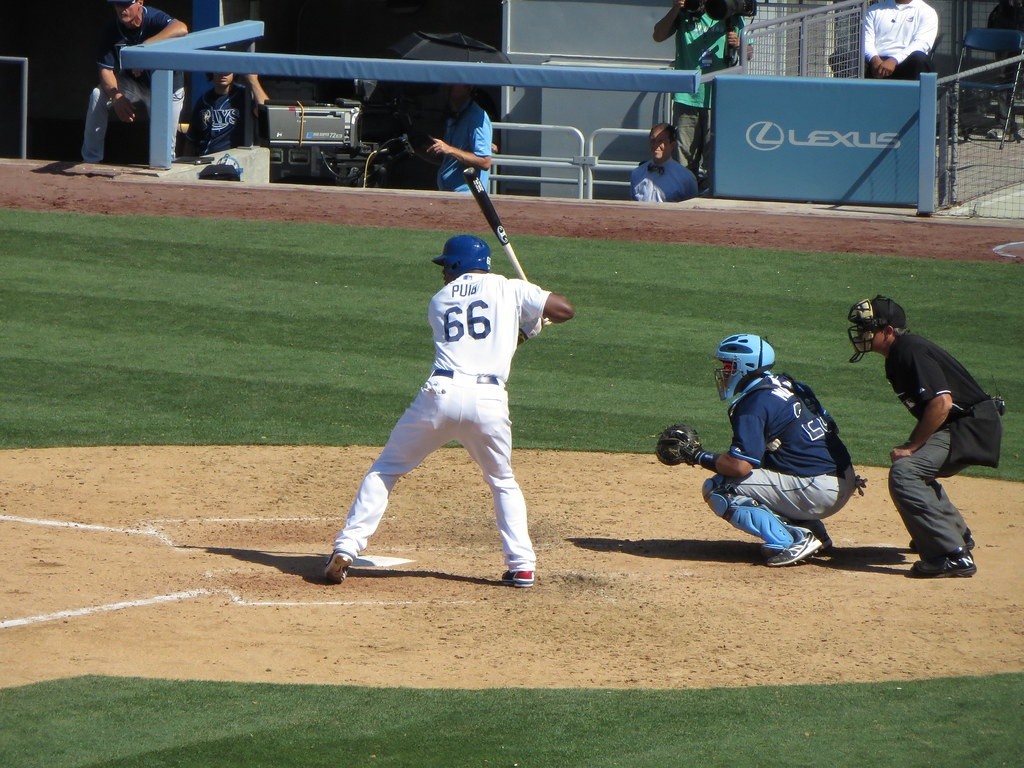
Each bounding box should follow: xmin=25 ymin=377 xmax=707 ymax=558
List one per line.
xmin=953 ymin=28 xmax=1024 ymax=149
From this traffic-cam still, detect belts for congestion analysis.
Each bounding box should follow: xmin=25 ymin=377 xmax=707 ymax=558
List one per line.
xmin=826 ymin=469 xmax=846 ymax=480
xmin=432 ymin=369 xmax=499 ymax=385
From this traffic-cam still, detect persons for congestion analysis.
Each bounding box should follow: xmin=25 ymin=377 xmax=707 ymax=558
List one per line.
xmin=631 ymin=123 xmax=698 ymax=203
xmin=862 ymin=0 xmax=940 ymax=81
xmin=184 ymin=70 xmax=269 ymax=155
xmin=427 ymin=83 xmax=498 ymax=192
xmin=82 ymin=0 xmax=189 ymax=164
xmin=322 ymin=235 xmax=574 ymax=587
xmin=655 ymin=333 xmax=855 ymax=567
xmin=653 ymin=0 xmax=754 ymax=198
xmin=848 ymin=294 xmax=1002 ymax=577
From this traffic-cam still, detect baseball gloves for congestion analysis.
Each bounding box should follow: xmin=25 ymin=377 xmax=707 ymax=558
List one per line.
xmin=655 ymin=424 xmax=700 ymax=466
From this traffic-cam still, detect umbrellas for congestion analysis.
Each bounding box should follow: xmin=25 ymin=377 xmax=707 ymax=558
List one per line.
xmin=389 ymin=30 xmax=511 ymax=65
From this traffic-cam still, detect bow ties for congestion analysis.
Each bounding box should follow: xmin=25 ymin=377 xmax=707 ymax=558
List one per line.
xmin=648 ymin=162 xmax=665 ymax=176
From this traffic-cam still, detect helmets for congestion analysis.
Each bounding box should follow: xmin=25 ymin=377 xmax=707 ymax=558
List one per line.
xmin=713 ymin=334 xmax=776 ymax=401
xmin=847 ymin=295 xmax=906 ymax=363
xmin=432 ymin=235 xmax=491 ymax=277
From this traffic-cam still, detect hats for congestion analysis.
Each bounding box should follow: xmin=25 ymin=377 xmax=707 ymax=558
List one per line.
xmin=108 ymin=0 xmax=136 ymax=4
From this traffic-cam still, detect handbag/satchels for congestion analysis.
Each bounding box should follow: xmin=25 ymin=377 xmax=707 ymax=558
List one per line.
xmin=949 ymin=416 xmax=1001 ymax=468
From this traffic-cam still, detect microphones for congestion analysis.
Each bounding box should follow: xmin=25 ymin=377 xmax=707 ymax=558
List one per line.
xmin=892 ymin=19 xmax=895 ymax=22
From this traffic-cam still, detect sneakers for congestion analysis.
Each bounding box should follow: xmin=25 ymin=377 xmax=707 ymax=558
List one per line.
xmin=909 ymin=528 xmax=975 ymax=550
xmin=987 ymin=122 xmax=1018 ymax=138
xmin=815 ymin=532 xmax=832 ymax=550
xmin=767 ymin=534 xmax=822 ymax=567
xmin=502 ymin=570 xmax=534 ymax=587
xmin=324 ymin=549 xmax=354 ymax=584
xmin=912 ymin=547 xmax=976 ymax=576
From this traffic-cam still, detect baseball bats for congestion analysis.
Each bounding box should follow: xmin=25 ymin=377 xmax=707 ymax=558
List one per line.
xmin=463 ymin=166 xmax=553 ymax=327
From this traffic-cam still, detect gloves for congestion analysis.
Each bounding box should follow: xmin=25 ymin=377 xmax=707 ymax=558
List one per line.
xmin=518 ymin=315 xmax=552 ymax=343
